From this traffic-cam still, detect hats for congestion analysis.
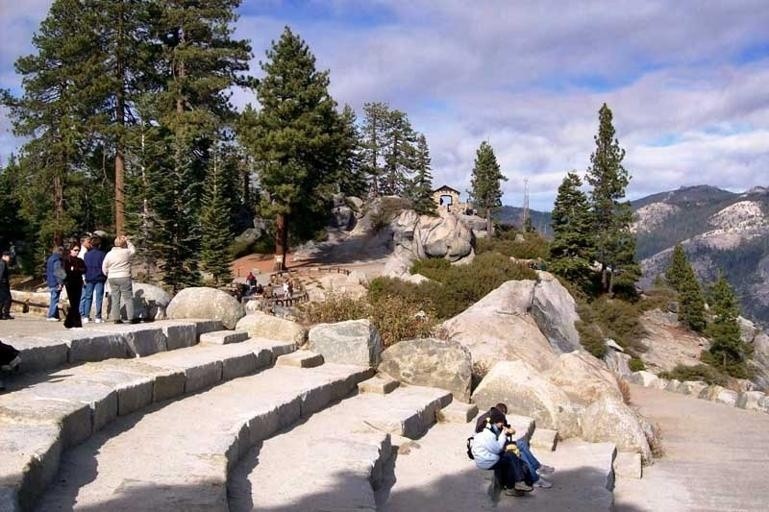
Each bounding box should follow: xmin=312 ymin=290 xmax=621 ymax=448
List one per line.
xmin=489 ymin=406 xmax=506 ymax=423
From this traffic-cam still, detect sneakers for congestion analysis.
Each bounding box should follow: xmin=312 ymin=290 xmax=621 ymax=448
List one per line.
xmin=505 ymin=487 xmax=523 ymax=496
xmin=43 ymin=312 xmax=144 ymax=335
xmin=513 ymin=481 xmax=532 ymax=492
xmin=538 ymin=463 xmax=555 ymax=475
xmin=534 ymin=478 xmax=552 ymax=489
xmin=0 ymin=313 xmax=15 ymax=320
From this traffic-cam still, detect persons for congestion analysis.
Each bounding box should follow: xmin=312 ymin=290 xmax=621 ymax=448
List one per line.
xmin=77 ymin=235 xmax=90 ymax=317
xmin=283 ymin=282 xmax=292 ymax=299
xmin=473 ymin=413 xmax=533 ymax=496
xmin=46 ymin=246 xmax=63 ymax=321
xmin=246 ymin=271 xmax=273 ymax=298
xmin=476 ymin=403 xmax=554 ymax=488
xmin=62 ymin=242 xmax=87 ymax=328
xmin=0 ymin=252 xmax=14 ymax=319
xmin=82 ymin=237 xmax=108 ymax=323
xmin=101 ymin=235 xmax=139 ymax=323
